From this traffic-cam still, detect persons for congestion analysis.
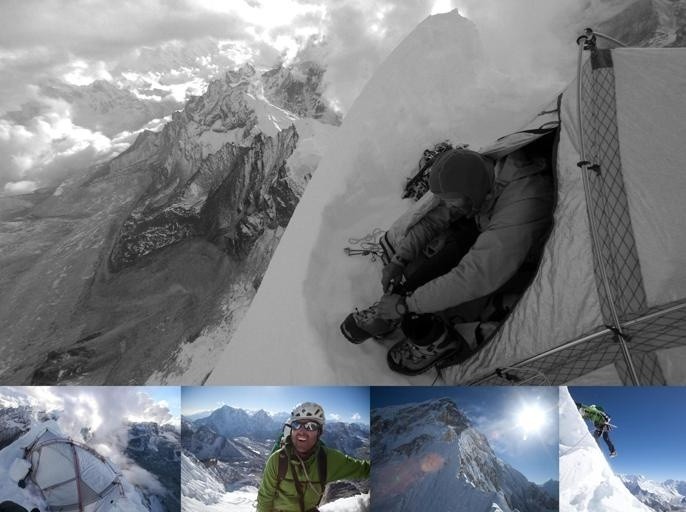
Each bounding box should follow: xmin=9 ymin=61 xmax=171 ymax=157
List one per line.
xmin=256 ymin=401 xmax=370 ymax=512
xmin=338 ymin=146 xmax=556 ymax=378
xmin=578 ymin=400 xmax=617 ymax=457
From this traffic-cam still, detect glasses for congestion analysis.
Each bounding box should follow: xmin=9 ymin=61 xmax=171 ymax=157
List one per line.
xmin=290 ymin=421 xmax=320 ymax=432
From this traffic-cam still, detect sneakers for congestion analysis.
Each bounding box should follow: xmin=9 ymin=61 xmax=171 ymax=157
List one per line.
xmin=610 ymin=451 xmax=617 ymax=457
xmin=340 ymin=303 xmax=401 ymax=344
xmin=387 ymin=326 xmax=463 ymax=376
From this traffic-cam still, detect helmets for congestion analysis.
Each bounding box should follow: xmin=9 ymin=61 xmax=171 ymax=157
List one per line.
xmin=289 ymin=401 xmax=325 ymax=425
xmin=579 ymin=407 xmax=585 ymax=417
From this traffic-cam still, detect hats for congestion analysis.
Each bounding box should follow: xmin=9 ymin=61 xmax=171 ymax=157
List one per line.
xmin=428 ymin=149 xmax=495 ymax=219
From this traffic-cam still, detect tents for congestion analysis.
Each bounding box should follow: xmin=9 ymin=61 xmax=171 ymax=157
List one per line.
xmin=437 ymin=24 xmax=686 ymax=384
xmin=19 ymin=427 xmax=125 ymax=512
